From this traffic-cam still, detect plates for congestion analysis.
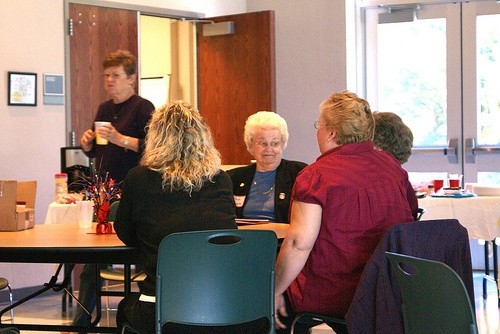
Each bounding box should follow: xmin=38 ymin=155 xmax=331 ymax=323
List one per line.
xmin=430 ymin=193 xmax=474 ymax=198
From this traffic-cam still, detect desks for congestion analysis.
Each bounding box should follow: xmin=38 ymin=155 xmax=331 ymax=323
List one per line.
xmin=44 ymin=201 xmax=94 ymax=317
xmin=0 ymin=221 xmax=290 ymax=334
xmin=417 ymin=195 xmax=500 ymax=308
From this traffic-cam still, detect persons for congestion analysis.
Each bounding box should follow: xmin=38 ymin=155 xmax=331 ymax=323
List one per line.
xmin=369 ymin=111 xmax=413 ymax=165
xmin=113 ymin=99 xmax=269 ymax=334
xmin=62 ymin=49 xmax=155 ymax=334
xmin=274 ymin=90 xmax=412 ymax=334
xmin=226 ymin=111 xmax=309 ymax=224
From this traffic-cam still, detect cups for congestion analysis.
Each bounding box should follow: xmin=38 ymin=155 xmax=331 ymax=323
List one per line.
xmin=94 ymin=122 xmax=111 ymax=145
xmin=449 ymin=179 xmax=460 ymax=187
xmin=434 ymin=179 xmax=444 ymax=193
xmin=75 ymin=201 xmax=93 ymax=227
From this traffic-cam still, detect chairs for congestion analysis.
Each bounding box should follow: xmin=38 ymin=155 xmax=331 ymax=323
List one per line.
xmin=385 ymin=252 xmax=478 ymax=334
xmin=289 ymin=219 xmax=476 ymax=334
xmin=122 ymin=229 xmax=278 ymax=334
xmin=0 ymin=277 xmax=14 ymax=324
xmin=91 ymin=264 xmax=147 ymax=328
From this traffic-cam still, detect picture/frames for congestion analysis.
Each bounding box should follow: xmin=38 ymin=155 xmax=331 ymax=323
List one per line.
xmin=7 ymin=71 xmax=37 ymax=106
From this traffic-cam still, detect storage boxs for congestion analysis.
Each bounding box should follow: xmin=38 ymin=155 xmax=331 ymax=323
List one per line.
xmin=0 ymin=180 xmax=38 ymax=232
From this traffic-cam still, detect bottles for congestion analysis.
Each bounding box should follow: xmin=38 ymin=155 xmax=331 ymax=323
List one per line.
xmin=55 ymin=173 xmax=67 ymax=204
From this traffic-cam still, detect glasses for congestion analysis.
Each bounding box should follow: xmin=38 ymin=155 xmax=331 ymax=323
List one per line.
xmin=252 ymin=140 xmax=283 ymax=147
xmin=313 ymin=121 xmax=333 ymax=129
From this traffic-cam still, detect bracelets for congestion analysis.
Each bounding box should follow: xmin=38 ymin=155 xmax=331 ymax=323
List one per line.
xmin=124 ymin=136 xmax=129 ymax=149
